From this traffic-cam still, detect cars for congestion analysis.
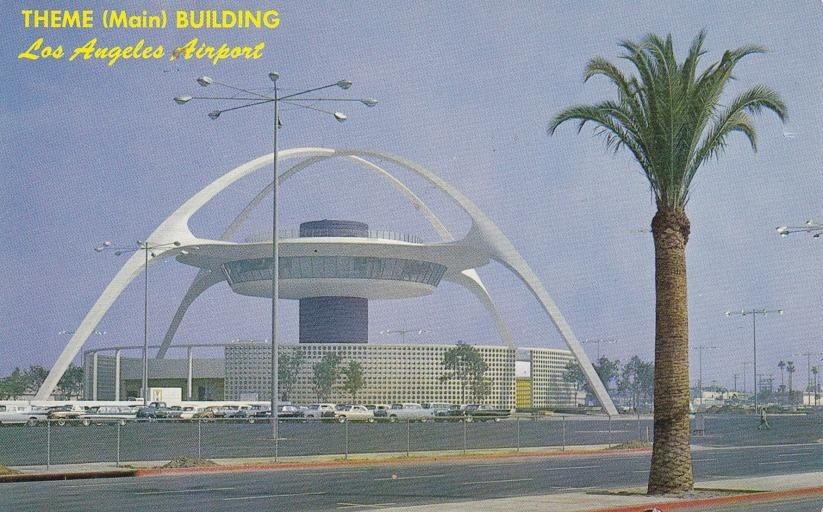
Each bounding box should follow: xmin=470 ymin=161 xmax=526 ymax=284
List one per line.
xmin=0 ymin=397 xmax=510 ymax=427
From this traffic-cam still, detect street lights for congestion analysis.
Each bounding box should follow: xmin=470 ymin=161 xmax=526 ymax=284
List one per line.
xmin=95 ymin=240 xmax=180 ymax=407
xmin=726 ymin=308 xmax=784 ymax=414
xmin=175 ymin=71 xmax=378 ymax=437
